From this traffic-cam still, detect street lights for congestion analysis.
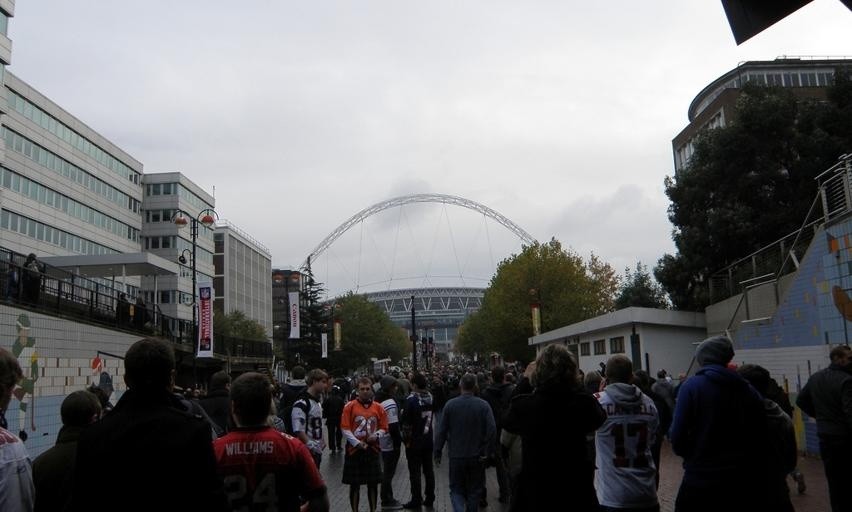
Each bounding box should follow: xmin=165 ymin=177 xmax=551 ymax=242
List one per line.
xmin=422 ymin=327 xmax=434 ymax=369
xmin=408 ymin=293 xmax=419 ymax=373
xmin=323 ymin=302 xmax=344 ymax=372
xmin=167 ymin=204 xmax=223 ymax=394
xmin=274 ymin=271 xmax=302 ymax=385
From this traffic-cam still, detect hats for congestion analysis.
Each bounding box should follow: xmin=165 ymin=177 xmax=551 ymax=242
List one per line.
xmin=382 ymin=375 xmax=395 ymax=390
xmin=695 ymin=336 xmax=734 ymax=366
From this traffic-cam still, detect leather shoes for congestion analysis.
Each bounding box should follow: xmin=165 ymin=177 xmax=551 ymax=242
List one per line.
xmin=403 ymin=501 xmax=422 ymax=512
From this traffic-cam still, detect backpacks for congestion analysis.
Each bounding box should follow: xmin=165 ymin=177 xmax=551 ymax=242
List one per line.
xmin=279 ymin=394 xmax=310 ymax=437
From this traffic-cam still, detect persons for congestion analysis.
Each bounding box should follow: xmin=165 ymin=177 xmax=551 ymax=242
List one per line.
xmin=212 ymin=371 xmax=332 ymax=511
xmin=74 ymin=338 xmax=225 ymax=510
xmin=115 ymin=291 xmax=133 ymax=323
xmin=11 ymin=314 xmax=39 ymax=442
xmin=88 ymin=357 xmax=119 ymax=409
xmin=24 ymin=251 xmax=46 ymax=303
xmin=175 ymin=338 xmax=807 ymax=511
xmin=31 ymin=389 xmax=104 ymax=512
xmin=795 ymin=343 xmax=851 ymax=510
xmin=2 ymin=345 xmax=37 ymax=512
xmin=135 ymin=296 xmax=150 ymax=326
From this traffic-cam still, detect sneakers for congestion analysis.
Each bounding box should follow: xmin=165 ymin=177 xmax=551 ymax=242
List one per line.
xmin=381 ymin=501 xmax=403 ymax=509
xmin=797 ymin=473 xmax=806 ymax=493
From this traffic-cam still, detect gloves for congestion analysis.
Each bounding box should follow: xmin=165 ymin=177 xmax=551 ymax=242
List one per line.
xmin=307 ymin=440 xmax=323 ymax=455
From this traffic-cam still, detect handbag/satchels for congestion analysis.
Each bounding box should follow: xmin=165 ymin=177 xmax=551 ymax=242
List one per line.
xmin=765 ymin=398 xmax=797 ymax=474
